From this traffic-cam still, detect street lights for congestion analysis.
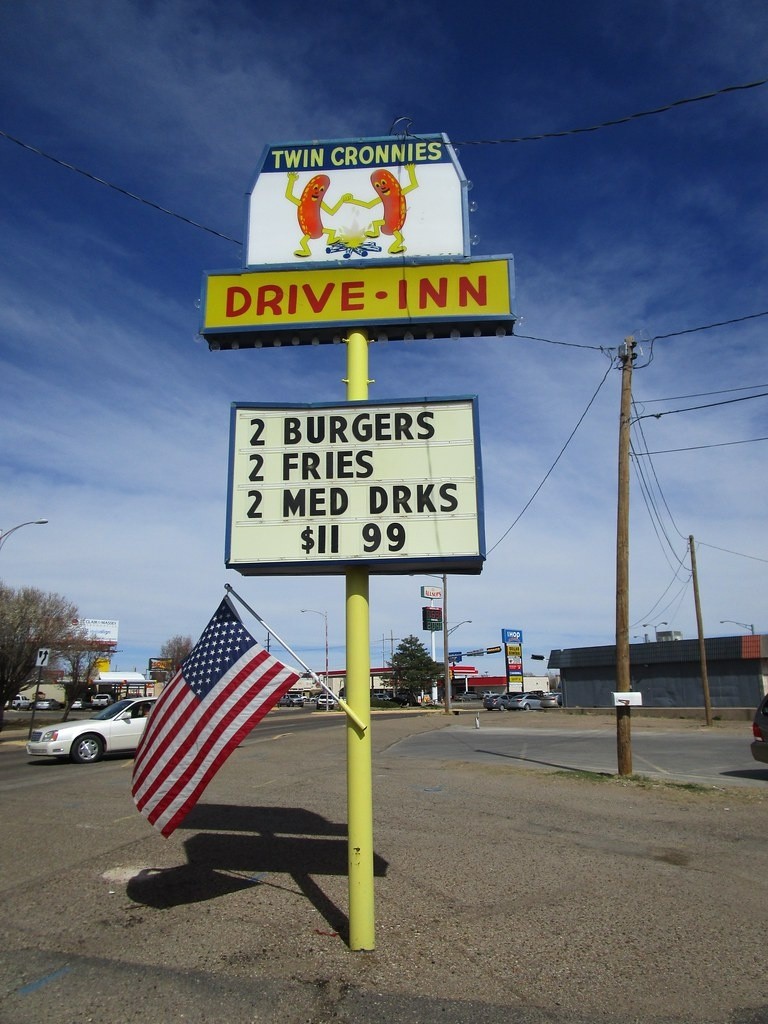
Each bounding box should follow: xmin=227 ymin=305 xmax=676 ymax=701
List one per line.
xmin=443 ymin=620 xmax=473 ymax=714
xmin=301 ymin=609 xmax=329 ymax=711
xmin=642 ymin=621 xmax=668 ymax=642
xmin=720 ymin=620 xmax=755 ymax=635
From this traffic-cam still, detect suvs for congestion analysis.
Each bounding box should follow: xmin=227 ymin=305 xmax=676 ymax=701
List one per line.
xmin=317 ymin=694 xmax=334 ymax=710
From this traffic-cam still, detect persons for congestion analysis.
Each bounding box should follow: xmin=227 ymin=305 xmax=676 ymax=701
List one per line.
xmin=142 ymin=703 xmax=151 ymax=717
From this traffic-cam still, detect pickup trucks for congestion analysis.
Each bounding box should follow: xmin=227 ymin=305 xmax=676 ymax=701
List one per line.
xmin=90 ymin=693 xmax=114 ymax=711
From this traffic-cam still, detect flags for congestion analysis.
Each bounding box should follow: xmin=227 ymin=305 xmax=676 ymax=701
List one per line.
xmin=132 ymin=593 xmax=302 ymax=840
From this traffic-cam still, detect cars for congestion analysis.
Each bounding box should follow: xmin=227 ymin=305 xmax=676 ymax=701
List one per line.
xmin=12 ymin=694 xmax=30 ymax=710
xmin=483 ymin=694 xmax=513 ymax=711
xmin=454 ymin=693 xmax=471 ymax=701
xmin=539 ymin=693 xmax=562 ymax=708
xmin=298 ymin=694 xmax=307 ymax=702
xmin=309 ymin=695 xmax=319 ymax=703
xmin=25 ymin=696 xmax=159 ymax=764
xmin=374 ymin=693 xmax=392 ymax=701
xmin=508 ymin=694 xmax=544 ymax=710
xmin=36 ymin=699 xmax=61 ymax=711
xmin=70 ymin=698 xmax=86 ymax=709
xmin=280 ymin=694 xmax=304 ymax=707
